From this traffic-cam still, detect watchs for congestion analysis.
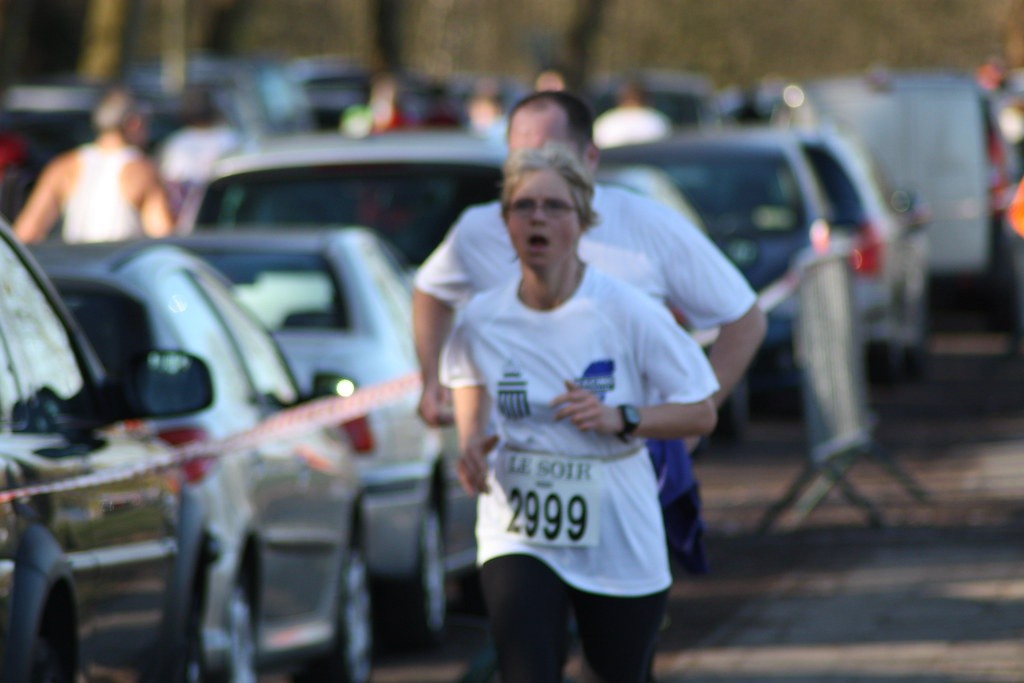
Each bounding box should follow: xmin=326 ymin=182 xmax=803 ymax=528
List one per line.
xmin=615 ymin=404 xmax=642 ymax=444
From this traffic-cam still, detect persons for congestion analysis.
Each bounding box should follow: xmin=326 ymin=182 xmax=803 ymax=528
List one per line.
xmin=410 ymin=91 xmax=770 ymax=577
xmin=592 ymin=74 xmax=672 ymax=149
xmin=147 ymin=86 xmax=249 ymax=221
xmin=439 ymin=141 xmax=721 ymax=683
xmin=11 ymin=84 xmax=176 ymax=248
xmin=334 ymin=65 xmax=569 ymax=143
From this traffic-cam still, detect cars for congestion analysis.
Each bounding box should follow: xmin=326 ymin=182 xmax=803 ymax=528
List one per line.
xmin=0 ymin=56 xmax=1001 ymax=474
xmin=19 ymin=240 xmax=389 ymax=682
xmin=179 ymin=220 xmax=483 ymax=657
xmin=1 ymin=225 xmax=237 ymax=682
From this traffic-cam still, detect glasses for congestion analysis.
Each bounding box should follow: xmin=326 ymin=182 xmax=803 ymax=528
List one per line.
xmin=507 ymin=197 xmax=578 ymax=219
